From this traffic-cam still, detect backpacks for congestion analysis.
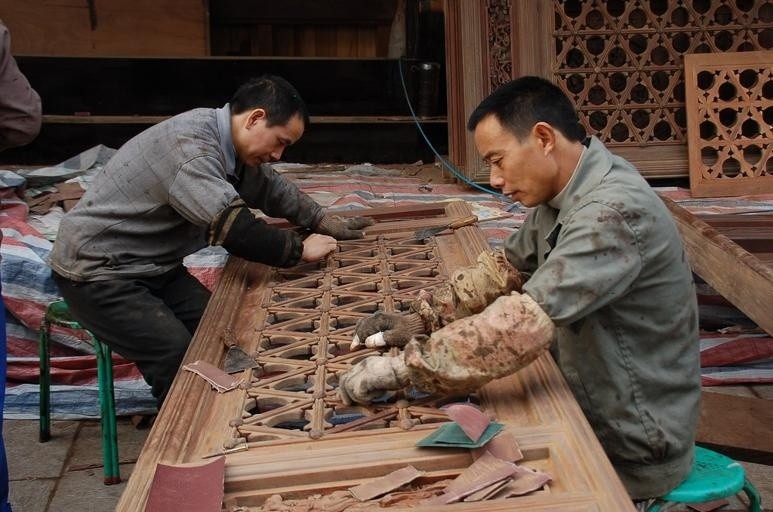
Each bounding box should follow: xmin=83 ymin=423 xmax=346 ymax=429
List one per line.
xmin=338 ymin=351 xmax=413 ymax=406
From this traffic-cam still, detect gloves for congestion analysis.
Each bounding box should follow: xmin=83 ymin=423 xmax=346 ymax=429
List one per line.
xmin=350 ymin=310 xmax=430 ymax=351
xmin=317 ymin=214 xmax=375 ymax=241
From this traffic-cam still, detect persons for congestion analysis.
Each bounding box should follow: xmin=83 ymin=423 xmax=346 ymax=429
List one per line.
xmin=44 ymin=76 xmax=375 ymax=414
xmin=333 ymin=70 xmax=705 ymax=505
xmin=0 ymin=26 xmax=45 ymax=511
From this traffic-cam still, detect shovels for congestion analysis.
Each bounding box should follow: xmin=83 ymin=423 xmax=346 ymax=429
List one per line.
xmin=222 ymin=328 xmax=260 ymax=374
xmin=415 ymin=215 xmax=477 ymax=241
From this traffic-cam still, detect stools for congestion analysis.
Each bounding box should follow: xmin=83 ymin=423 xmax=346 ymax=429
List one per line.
xmin=637 ymin=444 xmax=764 ymax=512
xmin=39 ymin=300 xmax=123 ymax=486
